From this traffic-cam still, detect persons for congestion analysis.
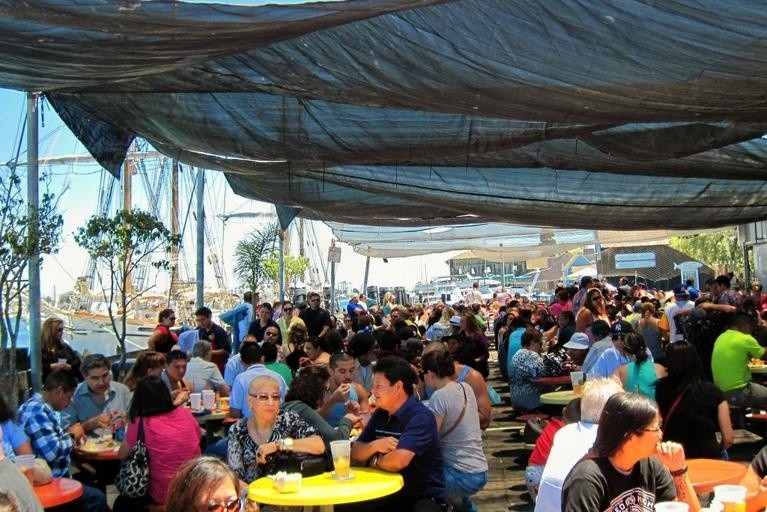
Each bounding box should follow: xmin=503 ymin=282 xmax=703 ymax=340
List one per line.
xmin=1 ymin=274 xmax=766 ymax=512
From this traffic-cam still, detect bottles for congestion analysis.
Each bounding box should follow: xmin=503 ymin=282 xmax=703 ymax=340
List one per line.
xmin=95 ymin=422 xmax=122 ymax=448
xmin=215 ymin=393 xmax=231 ymax=413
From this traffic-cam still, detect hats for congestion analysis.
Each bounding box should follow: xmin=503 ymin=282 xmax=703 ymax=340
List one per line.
xmin=563 ymin=332 xmax=589 ymax=350
xmin=673 ymin=285 xmax=690 ymax=296
xmin=610 ymin=320 xmax=632 ymax=337
xmin=350 ymin=293 xmax=358 ymax=301
xmin=448 ymin=315 xmax=461 ymax=325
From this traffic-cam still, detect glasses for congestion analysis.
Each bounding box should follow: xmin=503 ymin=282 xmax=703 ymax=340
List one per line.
xmin=208 ymin=498 xmax=241 ymax=512
xmin=249 ymin=393 xmax=280 ymax=402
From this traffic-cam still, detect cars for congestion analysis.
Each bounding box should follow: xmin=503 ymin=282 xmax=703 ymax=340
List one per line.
xmin=329 ymin=295 xmax=378 ymax=311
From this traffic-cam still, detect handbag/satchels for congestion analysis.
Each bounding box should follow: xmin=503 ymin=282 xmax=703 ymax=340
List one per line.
xmin=116 ymin=439 xmax=149 ymax=498
xmin=287 ymin=439 xmax=326 ymax=477
xmin=155 ymin=330 xmax=176 ymax=353
xmin=544 ymin=349 xmax=577 ymax=376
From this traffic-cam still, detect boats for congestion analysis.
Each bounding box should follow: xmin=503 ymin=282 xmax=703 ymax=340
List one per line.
xmin=400 ymin=261 xmax=537 ymax=307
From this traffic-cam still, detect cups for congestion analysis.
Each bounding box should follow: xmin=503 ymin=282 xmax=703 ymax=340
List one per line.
xmin=569 ymin=371 xmax=584 ymax=392
xmin=13 ymin=453 xmax=36 ymax=491
xmin=58 ymin=358 xmax=67 ymax=364
xmin=712 ymin=485 xmax=746 ymax=512
xmin=275 ymin=472 xmax=302 ymax=494
xmin=190 ymin=386 xmax=216 ymax=411
xmin=655 ymin=501 xmax=688 ymax=512
xmin=329 ymin=439 xmax=353 ymax=476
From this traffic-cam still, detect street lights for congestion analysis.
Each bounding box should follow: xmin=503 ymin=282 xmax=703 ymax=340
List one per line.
xmin=741 ymin=241 xmax=753 ymax=294
xmin=362 ymin=246 xmax=388 ymax=292
